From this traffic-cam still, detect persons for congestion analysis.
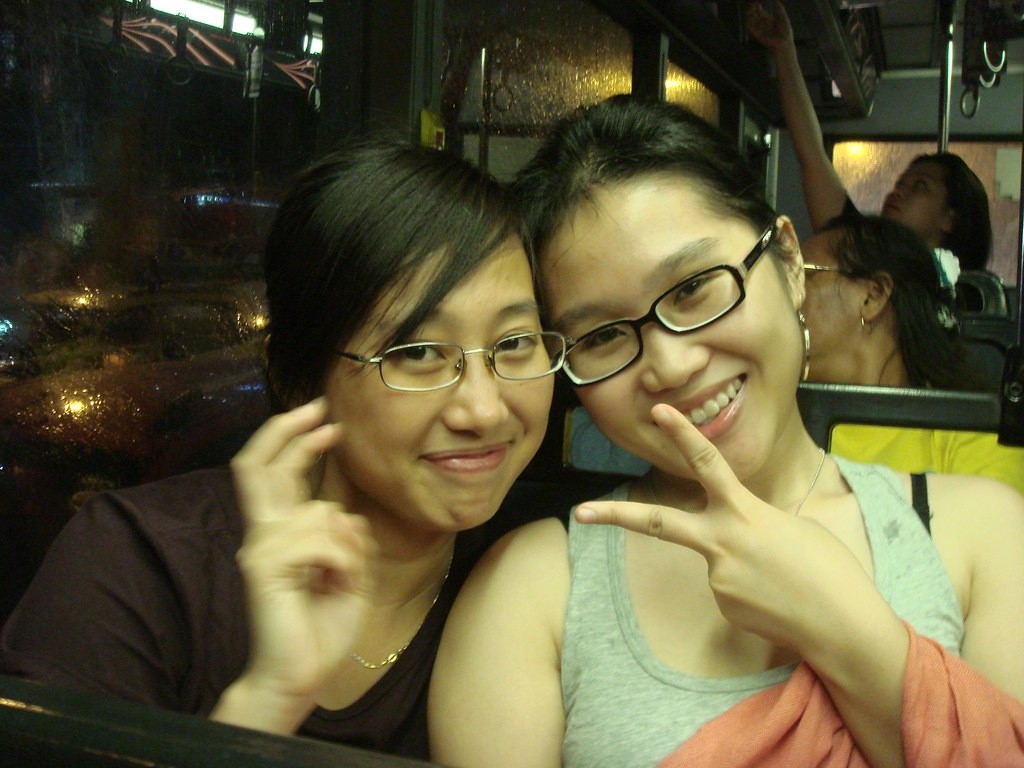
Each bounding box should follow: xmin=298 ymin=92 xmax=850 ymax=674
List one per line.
xmin=744 ymin=0 xmax=993 ymax=269
xmin=0 ymin=141 xmax=555 ymax=768
xmin=799 ymin=214 xmax=967 ymax=389
xmin=426 ymin=92 xmax=1024 ymax=768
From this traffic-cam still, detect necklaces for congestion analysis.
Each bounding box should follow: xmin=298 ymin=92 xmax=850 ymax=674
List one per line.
xmin=646 ymin=448 xmax=826 ymax=517
xmin=350 ymin=547 xmax=453 ymax=669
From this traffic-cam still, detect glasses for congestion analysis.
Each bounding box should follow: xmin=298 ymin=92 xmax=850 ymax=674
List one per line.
xmin=553 ymin=223 xmax=781 ymax=389
xmin=334 ymin=331 xmax=571 ymax=393
